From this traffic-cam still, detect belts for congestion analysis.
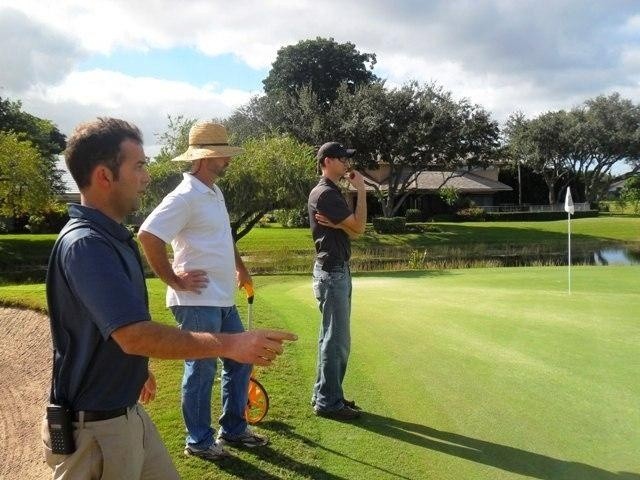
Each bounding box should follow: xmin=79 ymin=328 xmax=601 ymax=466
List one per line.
xmin=72 ymin=407 xmax=125 ymax=422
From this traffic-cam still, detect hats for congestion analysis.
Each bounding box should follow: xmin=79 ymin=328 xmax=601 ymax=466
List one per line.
xmin=170 ymin=122 xmax=247 ymax=162
xmin=316 ymin=141 xmax=357 ymax=163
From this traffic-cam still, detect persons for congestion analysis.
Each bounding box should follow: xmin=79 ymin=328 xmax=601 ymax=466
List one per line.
xmin=137 ymin=121 xmax=269 ymax=459
xmin=306 ymin=141 xmax=369 ymax=420
xmin=43 ymin=117 xmax=299 ymax=480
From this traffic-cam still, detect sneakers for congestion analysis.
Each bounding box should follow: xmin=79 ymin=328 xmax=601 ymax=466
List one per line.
xmin=311 ymin=396 xmax=354 ymax=407
xmin=313 ymin=406 xmax=360 ymax=420
xmin=184 ymin=439 xmax=228 ymax=460
xmin=217 ymin=426 xmax=268 ymax=448
